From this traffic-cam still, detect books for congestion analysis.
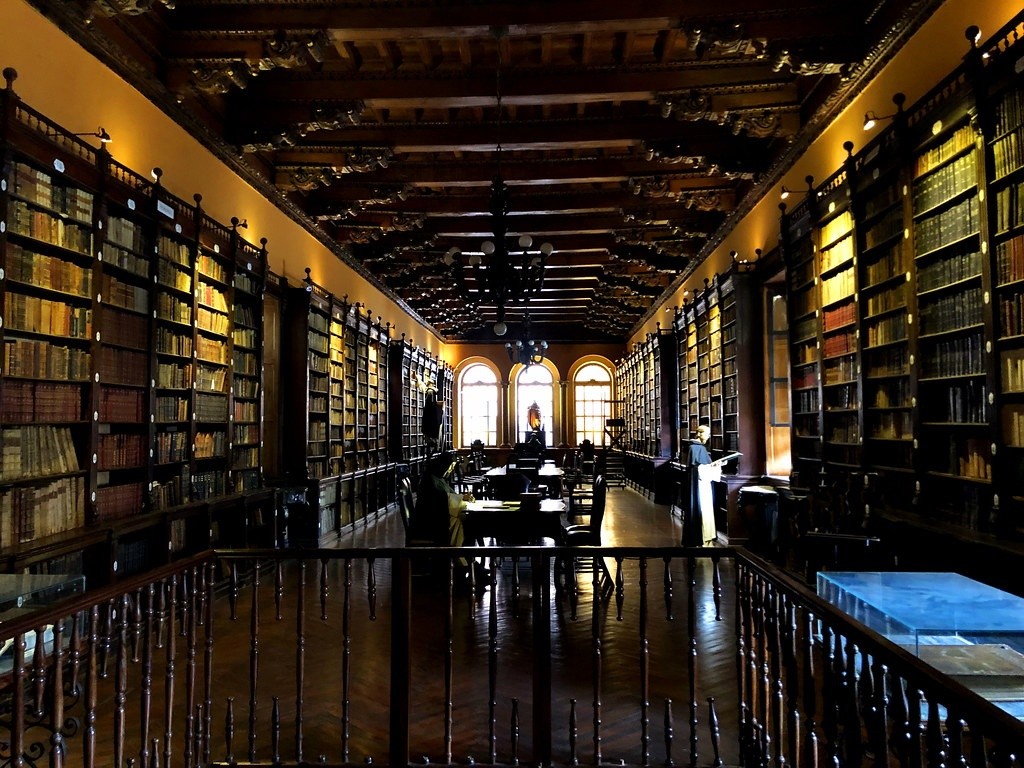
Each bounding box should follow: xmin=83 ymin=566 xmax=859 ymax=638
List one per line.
xmin=484 ymin=501 xmax=521 ymax=509
xmin=3 ymin=160 xmax=435 ymax=601
xmin=615 ymin=90 xmax=1024 ymax=480
xmin=717 ymin=452 xmax=743 ymax=463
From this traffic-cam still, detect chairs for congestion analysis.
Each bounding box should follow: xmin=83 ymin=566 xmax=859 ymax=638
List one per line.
xmin=394 ymin=439 xmax=615 ymax=594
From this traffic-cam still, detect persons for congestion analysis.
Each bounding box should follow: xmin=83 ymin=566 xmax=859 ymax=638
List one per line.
xmin=682 ymin=424 xmax=728 ymax=548
xmin=415 ymin=452 xmax=493 ymax=576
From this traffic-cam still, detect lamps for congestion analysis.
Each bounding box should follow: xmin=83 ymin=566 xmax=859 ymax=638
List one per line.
xmin=212 ymin=219 xmax=248 ymax=230
xmin=381 ymin=324 xmax=396 ymax=330
xmin=351 ymin=303 xmax=364 ymax=309
xmin=780 ymin=186 xmax=809 ymax=200
xmin=441 ymin=215 xmax=556 ymax=374
xmin=632 ymin=341 xmax=636 ymax=345
xmin=683 ymin=288 xmax=702 ymax=296
xmin=50 ymin=126 xmax=112 ymax=142
xmin=863 ymin=110 xmax=896 ymax=131
xmin=665 ymin=306 xmax=681 ymax=313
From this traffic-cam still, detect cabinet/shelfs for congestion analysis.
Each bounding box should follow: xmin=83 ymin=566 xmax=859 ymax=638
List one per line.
xmin=617 ymin=321 xmax=677 ymax=506
xmin=669 ymin=247 xmax=766 ymax=551
xmin=0 ymin=67 xmax=270 ymax=716
xmin=277 ymin=268 xmax=395 ymax=547
xmin=779 ymin=9 xmax=1024 ymax=599
xmin=437 ymin=360 xmax=457 ymax=458
xmin=390 ymin=333 xmax=440 ymax=505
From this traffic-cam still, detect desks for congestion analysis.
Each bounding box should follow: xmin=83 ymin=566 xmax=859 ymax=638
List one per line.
xmin=815 ymin=569 xmax=1024 ymax=720
xmin=484 ymin=464 xmax=565 ymax=497
xmin=460 ymin=498 xmax=570 ymax=594
